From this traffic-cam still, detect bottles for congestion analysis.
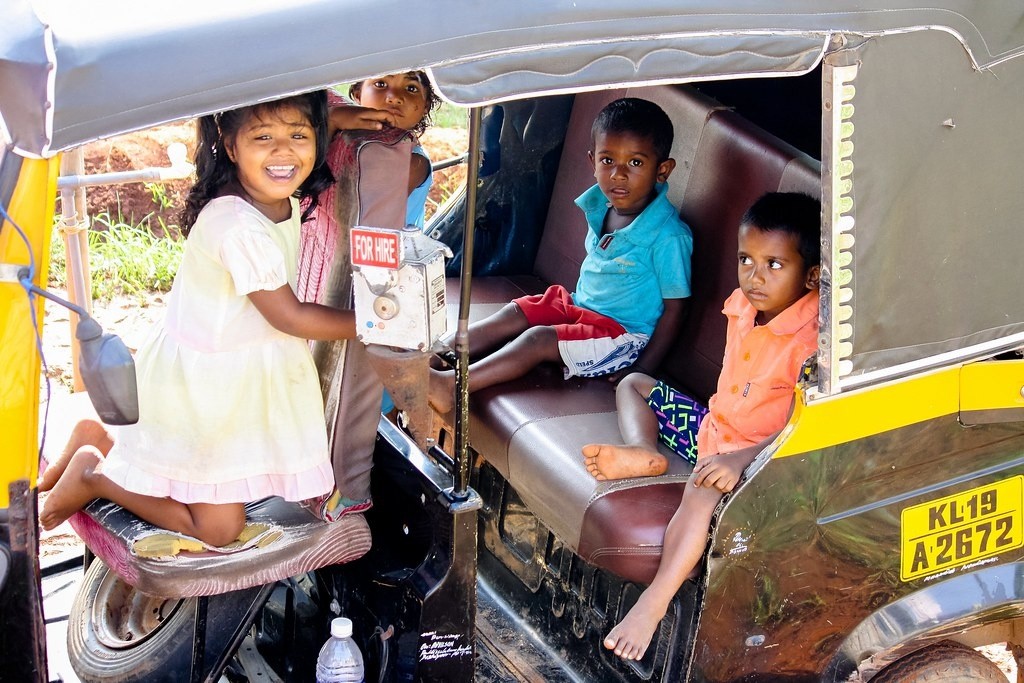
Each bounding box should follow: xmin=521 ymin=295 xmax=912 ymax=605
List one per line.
xmin=315 ymin=617 xmax=365 ymax=683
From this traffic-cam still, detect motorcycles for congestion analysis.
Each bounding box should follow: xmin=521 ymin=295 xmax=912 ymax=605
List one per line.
xmin=0 ymin=0 xmax=1024 ymax=682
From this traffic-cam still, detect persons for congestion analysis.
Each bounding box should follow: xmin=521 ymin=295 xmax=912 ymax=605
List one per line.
xmin=38 ymin=89 xmax=396 ymax=548
xmin=427 ymin=96 xmax=693 ymax=415
xmin=581 ymin=190 xmax=821 ymax=662
xmin=348 ymin=72 xmax=432 ymax=427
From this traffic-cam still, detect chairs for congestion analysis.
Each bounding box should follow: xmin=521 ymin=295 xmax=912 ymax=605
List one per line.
xmin=69 ymin=91 xmax=414 ymax=600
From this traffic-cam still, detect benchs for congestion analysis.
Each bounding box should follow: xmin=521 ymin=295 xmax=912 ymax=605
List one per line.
xmin=444 ymin=85 xmax=819 ymax=576
